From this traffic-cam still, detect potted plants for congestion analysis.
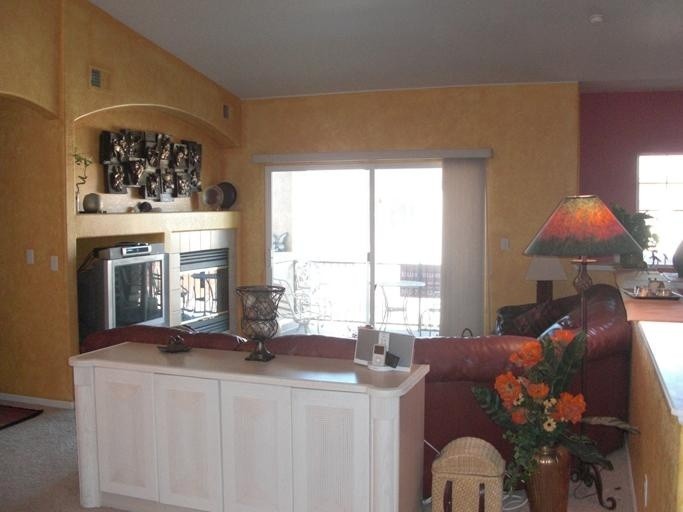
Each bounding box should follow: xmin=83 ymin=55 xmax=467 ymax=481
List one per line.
xmin=610 ymin=201 xmax=658 ymax=268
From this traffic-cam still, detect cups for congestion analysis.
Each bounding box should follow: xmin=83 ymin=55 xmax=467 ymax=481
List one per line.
xmin=634 ymin=285 xmax=648 ymax=295
xmin=656 ymin=288 xmax=673 ymax=297
xmin=659 ymin=272 xmax=678 ymax=290
xmin=637 ymin=288 xmax=652 ymax=297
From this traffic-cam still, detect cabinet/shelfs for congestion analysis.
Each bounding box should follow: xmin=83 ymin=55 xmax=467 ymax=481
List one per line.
xmin=221 ymin=379 xmax=371 ymax=512
xmin=77 ymin=211 xmax=241 ymax=238
xmin=624 ymin=322 xmax=682 ymax=512
xmin=94 ymin=368 xmax=223 ymax=512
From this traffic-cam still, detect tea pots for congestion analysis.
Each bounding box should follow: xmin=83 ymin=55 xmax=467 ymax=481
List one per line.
xmin=648 ymin=277 xmax=665 ymax=294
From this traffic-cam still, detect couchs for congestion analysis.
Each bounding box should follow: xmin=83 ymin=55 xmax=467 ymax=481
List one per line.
xmin=235 ymin=283 xmax=544 ymax=497
xmin=80 ymin=324 xmax=245 ymax=353
xmin=494 ymin=284 xmax=629 ymax=456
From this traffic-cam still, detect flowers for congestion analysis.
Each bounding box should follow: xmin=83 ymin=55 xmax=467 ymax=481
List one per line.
xmin=470 ymin=330 xmax=640 ymax=495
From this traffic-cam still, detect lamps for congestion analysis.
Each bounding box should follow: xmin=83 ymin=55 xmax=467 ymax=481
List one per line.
xmin=524 ymin=193 xmax=643 ymax=511
xmin=234 ymin=283 xmax=285 ymax=363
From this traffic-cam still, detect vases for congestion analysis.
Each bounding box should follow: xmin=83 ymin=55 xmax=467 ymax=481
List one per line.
xmin=523 ymin=444 xmax=570 ymax=511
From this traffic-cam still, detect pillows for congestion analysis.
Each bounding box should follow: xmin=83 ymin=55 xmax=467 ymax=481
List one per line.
xmin=513 ymin=294 xmax=563 ymax=338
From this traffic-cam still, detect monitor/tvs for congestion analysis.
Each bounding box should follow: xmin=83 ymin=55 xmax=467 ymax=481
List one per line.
xmin=75 ymin=255 xmax=165 ymax=335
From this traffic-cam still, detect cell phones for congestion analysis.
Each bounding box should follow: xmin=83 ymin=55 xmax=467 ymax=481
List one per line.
xmin=372 ymin=342 xmax=386 ymax=367
